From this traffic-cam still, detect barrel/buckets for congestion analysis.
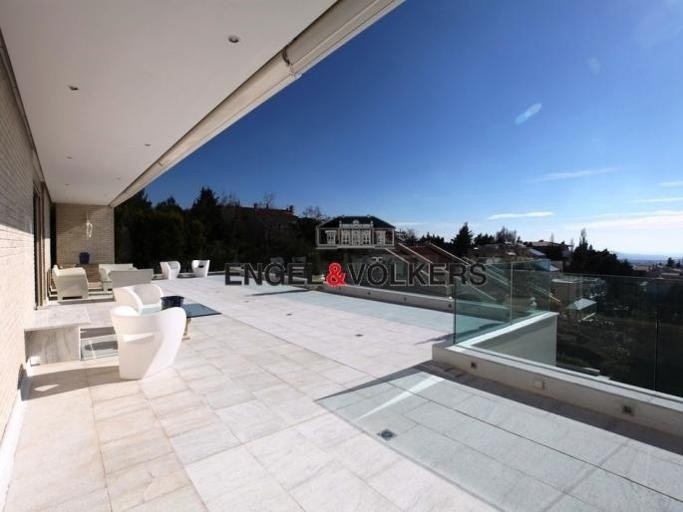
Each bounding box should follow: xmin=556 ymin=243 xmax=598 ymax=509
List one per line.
xmin=79 ymin=252 xmax=90 ymax=265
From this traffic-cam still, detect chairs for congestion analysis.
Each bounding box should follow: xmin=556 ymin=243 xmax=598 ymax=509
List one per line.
xmin=109 ymin=283 xmax=186 ymax=380
xmin=159 ymin=259 xmax=210 ymax=280
xmin=51 ymin=263 xmax=89 ymax=301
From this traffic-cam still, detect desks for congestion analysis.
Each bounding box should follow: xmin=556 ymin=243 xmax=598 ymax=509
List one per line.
xmin=23 ymin=304 xmax=90 ymax=365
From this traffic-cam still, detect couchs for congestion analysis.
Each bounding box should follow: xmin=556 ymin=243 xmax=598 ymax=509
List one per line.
xmin=97 ymin=263 xmax=154 ymax=300
